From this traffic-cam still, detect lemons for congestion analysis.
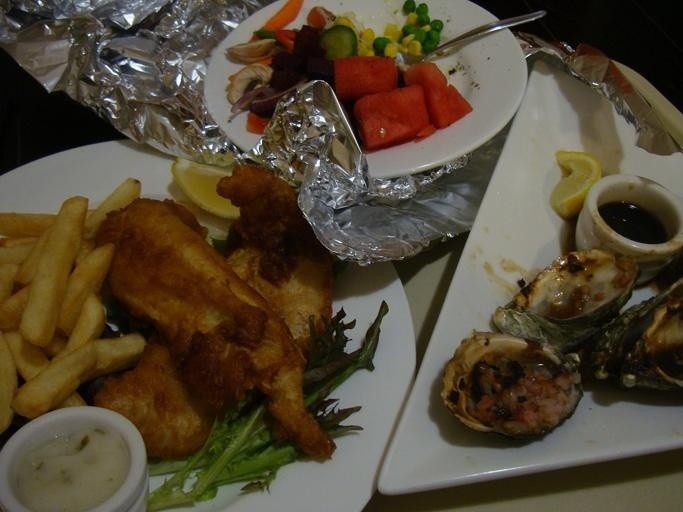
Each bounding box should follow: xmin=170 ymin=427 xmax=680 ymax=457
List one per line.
xmin=170 ymin=156 xmax=245 ymax=220
xmin=550 ymin=150 xmax=600 ymax=220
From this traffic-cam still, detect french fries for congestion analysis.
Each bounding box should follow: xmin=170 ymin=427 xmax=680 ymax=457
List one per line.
xmin=0 ymin=177 xmax=147 ymax=434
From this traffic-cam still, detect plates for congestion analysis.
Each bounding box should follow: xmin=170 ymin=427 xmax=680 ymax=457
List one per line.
xmin=370 ymin=53 xmax=682 ymax=494
xmin=202 ymin=0 xmax=529 ymax=179
xmin=1 ymin=136 xmax=419 ymax=512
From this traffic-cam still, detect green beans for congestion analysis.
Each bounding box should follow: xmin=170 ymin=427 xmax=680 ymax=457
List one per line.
xmin=373 ymin=0 xmax=443 ymax=57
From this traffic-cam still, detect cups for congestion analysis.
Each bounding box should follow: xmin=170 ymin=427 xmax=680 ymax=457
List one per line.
xmin=577 ymin=172 xmax=683 ymax=286
xmin=0 ymin=405 xmax=152 ymax=512
